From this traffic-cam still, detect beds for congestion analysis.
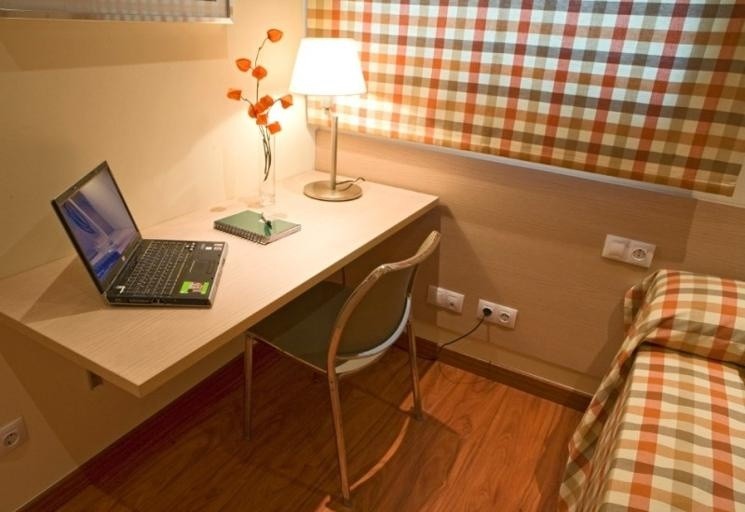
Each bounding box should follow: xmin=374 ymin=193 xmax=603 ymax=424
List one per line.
xmin=558 ymin=268 xmax=745 ymax=511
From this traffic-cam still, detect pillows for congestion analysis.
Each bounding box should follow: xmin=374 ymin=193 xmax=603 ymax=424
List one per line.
xmin=636 ymin=268 xmax=745 ymax=367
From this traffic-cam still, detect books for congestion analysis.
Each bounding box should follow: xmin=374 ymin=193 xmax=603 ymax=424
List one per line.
xmin=214 ymin=210 xmax=302 ymax=245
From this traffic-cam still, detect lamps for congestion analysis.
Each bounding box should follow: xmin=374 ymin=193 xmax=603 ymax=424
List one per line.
xmin=288 ymin=37 xmax=368 ymax=201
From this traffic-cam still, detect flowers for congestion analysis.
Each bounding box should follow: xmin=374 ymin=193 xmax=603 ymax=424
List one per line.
xmin=224 ymin=27 xmax=295 ymax=184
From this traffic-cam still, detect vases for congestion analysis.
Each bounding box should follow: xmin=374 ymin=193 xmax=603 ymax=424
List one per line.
xmin=246 ymin=130 xmax=277 ymax=208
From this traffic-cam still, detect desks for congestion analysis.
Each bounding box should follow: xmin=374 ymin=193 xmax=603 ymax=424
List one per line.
xmin=0 ymin=169 xmax=439 ymax=399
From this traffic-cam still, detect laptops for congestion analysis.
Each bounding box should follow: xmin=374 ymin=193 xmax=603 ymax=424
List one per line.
xmin=50 ymin=161 xmax=228 ymax=308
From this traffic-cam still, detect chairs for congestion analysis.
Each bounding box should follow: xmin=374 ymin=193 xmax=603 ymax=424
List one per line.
xmin=242 ymin=229 xmax=444 ymax=502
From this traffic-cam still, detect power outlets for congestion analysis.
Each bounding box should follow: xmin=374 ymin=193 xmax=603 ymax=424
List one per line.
xmin=474 ymin=297 xmax=520 ymax=329
xmin=624 ymin=239 xmax=658 ymax=270
xmin=0 ymin=416 xmax=30 ymax=456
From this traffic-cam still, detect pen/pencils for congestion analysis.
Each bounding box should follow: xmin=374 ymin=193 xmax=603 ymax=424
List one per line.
xmin=262 ymin=215 xmax=273 ymax=229
